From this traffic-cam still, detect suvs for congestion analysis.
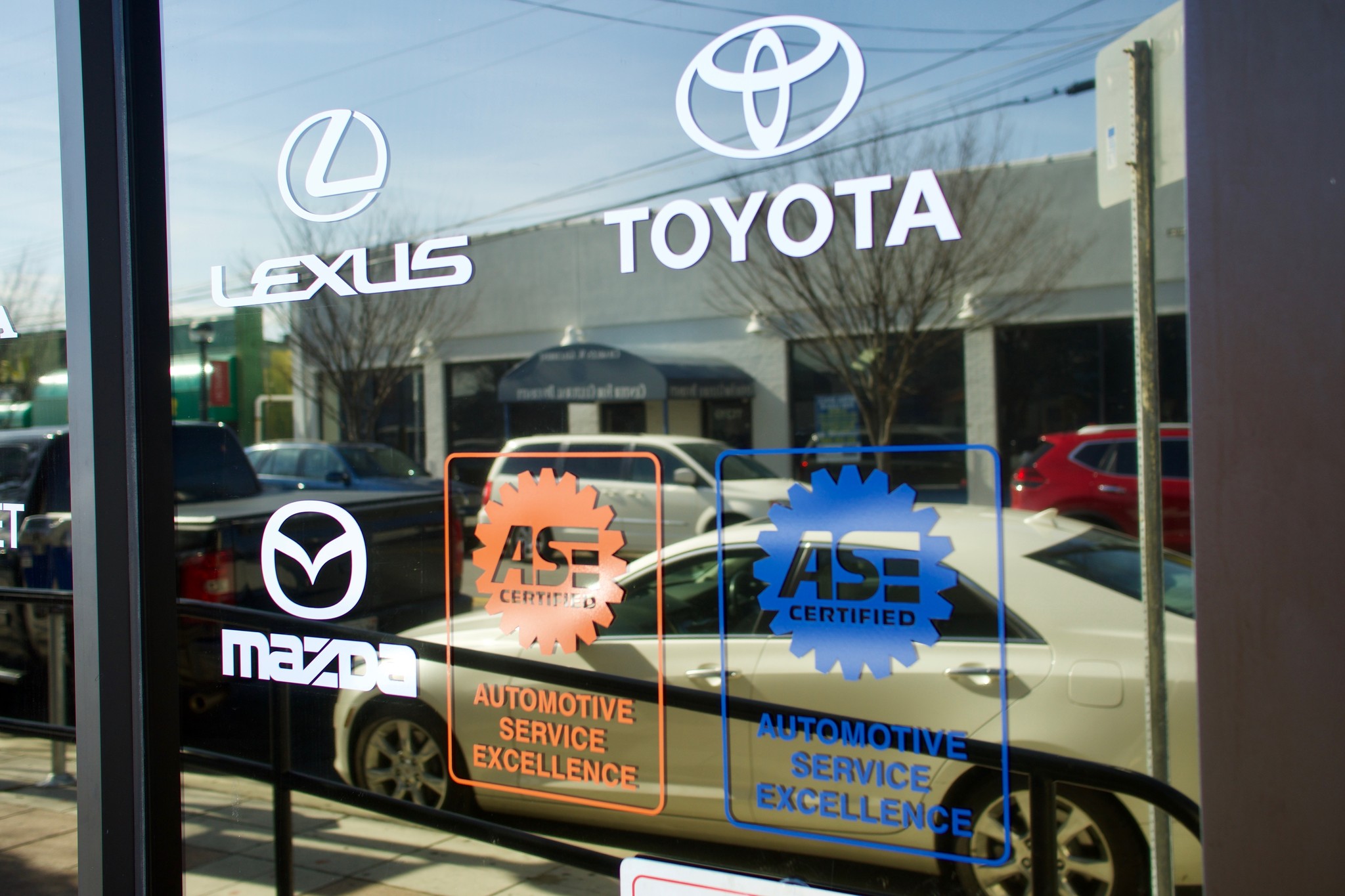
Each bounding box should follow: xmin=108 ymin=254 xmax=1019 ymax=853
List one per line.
xmin=1011 ymin=423 xmax=1195 ymax=548
xmin=481 ymin=431 xmax=812 ymax=571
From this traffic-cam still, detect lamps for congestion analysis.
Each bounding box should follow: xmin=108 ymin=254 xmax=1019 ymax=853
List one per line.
xmin=558 ymin=322 xmax=583 ymax=349
xmin=955 ymin=293 xmax=984 ymax=324
xmin=408 ymin=338 xmax=434 ymax=358
xmin=744 ymin=309 xmax=769 ymax=336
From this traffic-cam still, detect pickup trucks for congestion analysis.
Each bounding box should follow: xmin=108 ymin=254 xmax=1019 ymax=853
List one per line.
xmin=1 ymin=417 xmax=471 ymax=717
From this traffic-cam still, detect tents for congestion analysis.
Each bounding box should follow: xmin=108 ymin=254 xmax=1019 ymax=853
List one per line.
xmin=495 ymin=339 xmax=756 ymax=450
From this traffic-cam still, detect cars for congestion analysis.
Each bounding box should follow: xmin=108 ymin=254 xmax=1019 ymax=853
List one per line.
xmin=239 ymin=436 xmax=467 ymax=532
xmin=361 ymin=498 xmax=1206 ymax=896
